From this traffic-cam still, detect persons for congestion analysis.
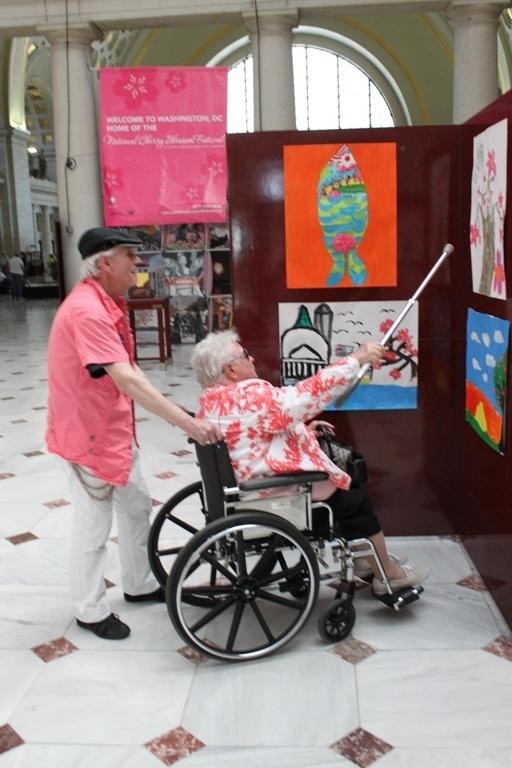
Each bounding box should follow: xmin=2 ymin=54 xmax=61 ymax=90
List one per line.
xmin=190 ymin=328 xmax=430 ymax=596
xmin=19 ymin=248 xmax=43 ymax=276
xmin=46 ymin=253 xmax=58 ymax=280
xmin=0 ymin=248 xmax=10 ymax=296
xmin=43 ymin=227 xmax=226 ymax=640
xmin=7 ymin=251 xmax=26 ymax=303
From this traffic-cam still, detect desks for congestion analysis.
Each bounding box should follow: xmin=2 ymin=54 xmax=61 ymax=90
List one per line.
xmin=124 ymin=295 xmax=173 ymax=363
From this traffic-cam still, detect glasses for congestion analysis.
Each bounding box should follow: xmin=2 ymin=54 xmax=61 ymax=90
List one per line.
xmin=106 ymin=250 xmax=137 ymax=261
xmin=228 ymin=348 xmax=250 ymax=366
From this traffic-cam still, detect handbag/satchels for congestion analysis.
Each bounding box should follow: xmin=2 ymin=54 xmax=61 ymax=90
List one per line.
xmin=317 ymin=435 xmax=369 ymax=488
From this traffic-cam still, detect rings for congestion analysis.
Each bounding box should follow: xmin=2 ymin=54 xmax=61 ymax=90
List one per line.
xmin=205 ymin=440 xmax=212 ymax=446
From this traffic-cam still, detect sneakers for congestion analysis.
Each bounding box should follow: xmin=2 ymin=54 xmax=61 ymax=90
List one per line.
xmin=75 ymin=611 xmax=131 ymax=639
xmin=122 ymin=587 xmax=168 ymax=603
xmin=351 ymin=549 xmax=411 ymax=578
xmin=372 ymin=563 xmax=430 ymax=597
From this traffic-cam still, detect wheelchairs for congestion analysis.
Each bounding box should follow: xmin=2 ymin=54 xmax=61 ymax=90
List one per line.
xmin=146 ymin=409 xmax=427 ymax=665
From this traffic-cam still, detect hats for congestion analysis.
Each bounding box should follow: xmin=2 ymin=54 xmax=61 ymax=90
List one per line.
xmin=77 ymin=226 xmax=146 ymax=260
